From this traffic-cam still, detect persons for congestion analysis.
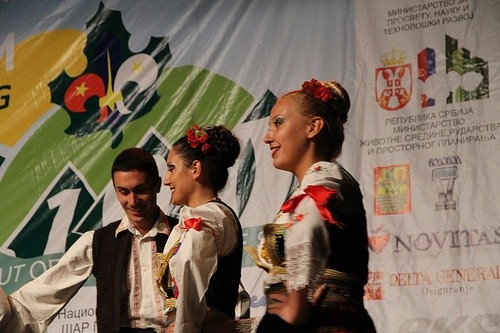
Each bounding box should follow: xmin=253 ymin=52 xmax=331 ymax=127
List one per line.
xmin=257 ymin=77 xmax=380 ymax=333
xmin=153 ymin=124 xmax=245 ymax=333
xmin=4 ymin=146 xmax=178 ymax=332
